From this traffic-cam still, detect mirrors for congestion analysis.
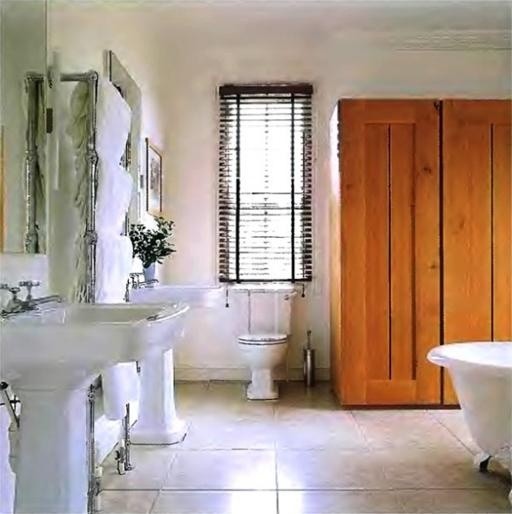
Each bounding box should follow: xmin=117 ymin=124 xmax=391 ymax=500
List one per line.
xmin=0 ymin=0 xmax=47 ymax=255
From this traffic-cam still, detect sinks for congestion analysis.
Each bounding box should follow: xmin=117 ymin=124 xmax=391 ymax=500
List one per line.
xmin=0 ymin=300 xmax=192 ymax=361
xmin=130 ymin=281 xmax=224 ymax=310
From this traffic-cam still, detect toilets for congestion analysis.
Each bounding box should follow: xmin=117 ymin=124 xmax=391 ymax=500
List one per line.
xmin=230 ymin=282 xmax=298 ymax=401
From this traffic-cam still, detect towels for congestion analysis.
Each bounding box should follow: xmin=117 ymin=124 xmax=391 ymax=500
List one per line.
xmin=20 ymin=70 xmax=47 ymax=255
xmin=65 ymin=71 xmax=140 ymax=420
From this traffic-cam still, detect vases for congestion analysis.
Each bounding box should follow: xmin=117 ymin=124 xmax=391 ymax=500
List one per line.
xmin=142 ymin=262 xmax=156 ymax=286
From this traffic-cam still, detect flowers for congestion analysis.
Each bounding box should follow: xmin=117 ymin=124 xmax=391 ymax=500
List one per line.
xmin=127 ymin=216 xmax=177 ymax=268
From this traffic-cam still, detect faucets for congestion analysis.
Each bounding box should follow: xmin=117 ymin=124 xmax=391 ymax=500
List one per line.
xmin=19 ymin=280 xmax=63 ymax=305
xmin=135 ymin=272 xmax=159 ymax=288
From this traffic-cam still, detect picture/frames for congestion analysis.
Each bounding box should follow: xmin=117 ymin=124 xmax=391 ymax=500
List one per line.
xmin=146 ymin=136 xmax=163 ymax=217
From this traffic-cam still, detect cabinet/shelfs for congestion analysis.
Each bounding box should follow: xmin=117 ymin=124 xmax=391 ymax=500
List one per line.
xmin=329 ymin=98 xmax=512 ymax=409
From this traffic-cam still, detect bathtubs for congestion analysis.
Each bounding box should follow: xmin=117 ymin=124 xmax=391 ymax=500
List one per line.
xmin=427 ymin=341 xmax=512 ymax=506
